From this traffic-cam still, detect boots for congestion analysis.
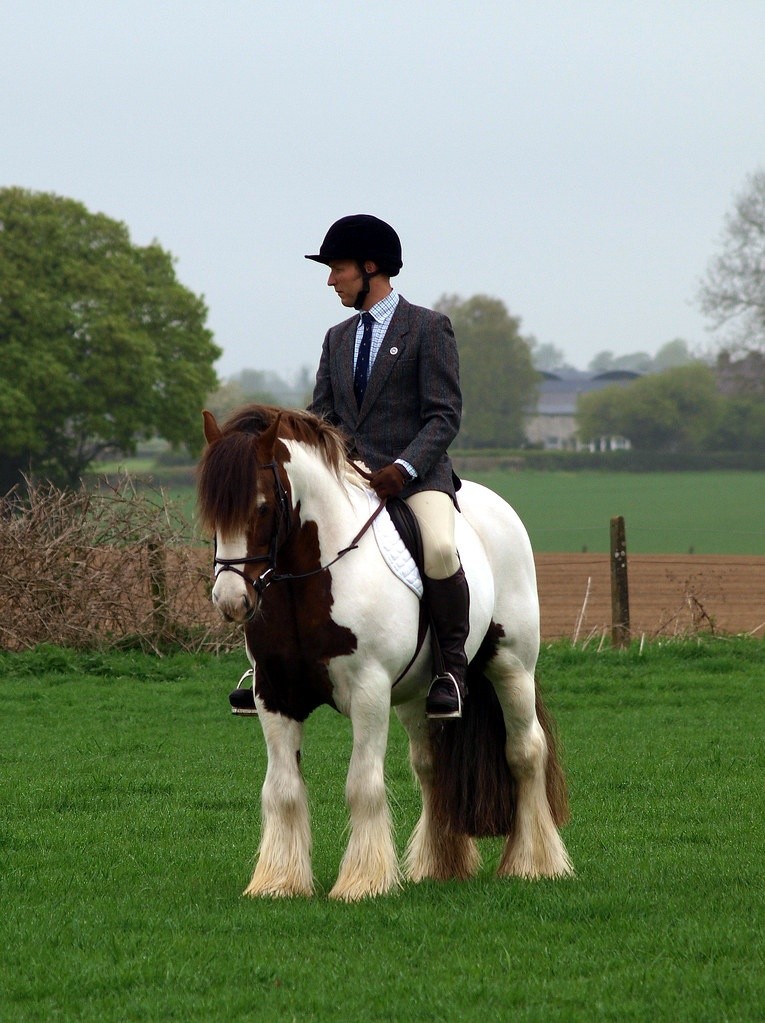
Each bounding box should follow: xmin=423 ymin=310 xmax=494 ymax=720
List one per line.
xmin=422 ymin=565 xmax=470 ymax=712
xmin=229 ymin=686 xmax=255 ymax=708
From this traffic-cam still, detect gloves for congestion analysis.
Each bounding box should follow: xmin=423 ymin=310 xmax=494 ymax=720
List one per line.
xmin=370 ymin=465 xmax=404 ymax=497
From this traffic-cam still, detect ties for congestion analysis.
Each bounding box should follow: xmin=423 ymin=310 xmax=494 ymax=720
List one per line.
xmin=353 ymin=312 xmax=374 ymax=414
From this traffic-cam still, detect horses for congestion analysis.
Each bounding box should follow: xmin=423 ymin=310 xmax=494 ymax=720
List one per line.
xmin=201 ymin=405 xmax=573 ymax=900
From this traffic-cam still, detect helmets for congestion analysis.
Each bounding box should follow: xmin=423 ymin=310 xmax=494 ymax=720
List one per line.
xmin=304 ymin=213 xmax=404 ymax=268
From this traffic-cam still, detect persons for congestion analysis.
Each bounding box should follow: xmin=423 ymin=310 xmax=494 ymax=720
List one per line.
xmin=228 ymin=214 xmax=471 ymax=714
